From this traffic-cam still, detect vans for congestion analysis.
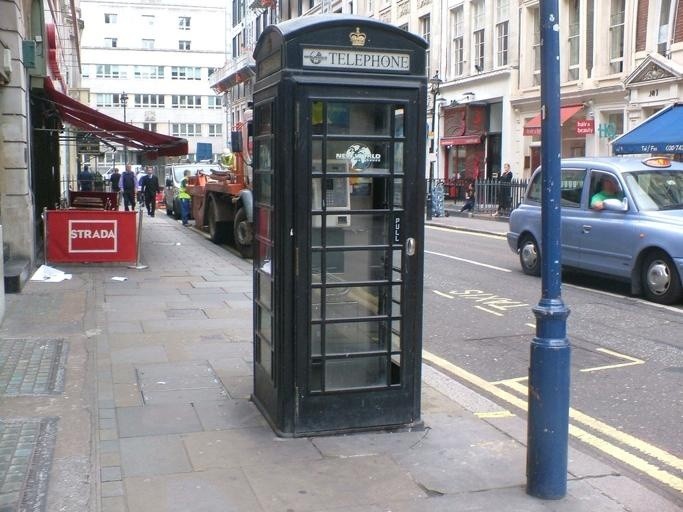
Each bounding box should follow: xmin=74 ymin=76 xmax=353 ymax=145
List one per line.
xmin=163 ymin=160 xmax=221 ymax=220
xmin=101 ymin=164 xmax=144 ymax=186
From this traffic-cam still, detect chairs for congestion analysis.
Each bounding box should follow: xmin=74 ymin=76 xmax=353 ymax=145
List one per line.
xmin=55 ymin=196 xmax=115 ymax=210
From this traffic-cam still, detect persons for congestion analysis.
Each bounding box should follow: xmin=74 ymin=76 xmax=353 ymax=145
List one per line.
xmin=589 ymin=174 xmax=625 ymax=212
xmin=491 ymin=164 xmax=513 ymax=217
xmin=459 ymin=182 xmax=476 ymax=213
xmin=178 ymin=169 xmax=195 ymax=227
xmin=79 ymin=163 xmax=160 ymax=217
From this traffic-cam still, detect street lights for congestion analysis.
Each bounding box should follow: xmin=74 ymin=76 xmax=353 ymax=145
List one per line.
xmin=426 ymin=70 xmax=441 ymax=221
xmin=119 ymin=91 xmax=128 ymax=172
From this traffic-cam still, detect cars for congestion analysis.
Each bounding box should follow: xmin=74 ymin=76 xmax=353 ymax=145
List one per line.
xmin=506 ymin=156 xmax=682 ymax=305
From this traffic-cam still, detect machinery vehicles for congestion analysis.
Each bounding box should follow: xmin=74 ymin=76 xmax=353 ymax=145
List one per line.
xmin=182 ymin=110 xmax=253 ymax=259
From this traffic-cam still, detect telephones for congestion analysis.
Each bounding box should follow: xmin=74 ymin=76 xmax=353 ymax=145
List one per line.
xmin=311 ymin=159 xmax=350 ymax=228
